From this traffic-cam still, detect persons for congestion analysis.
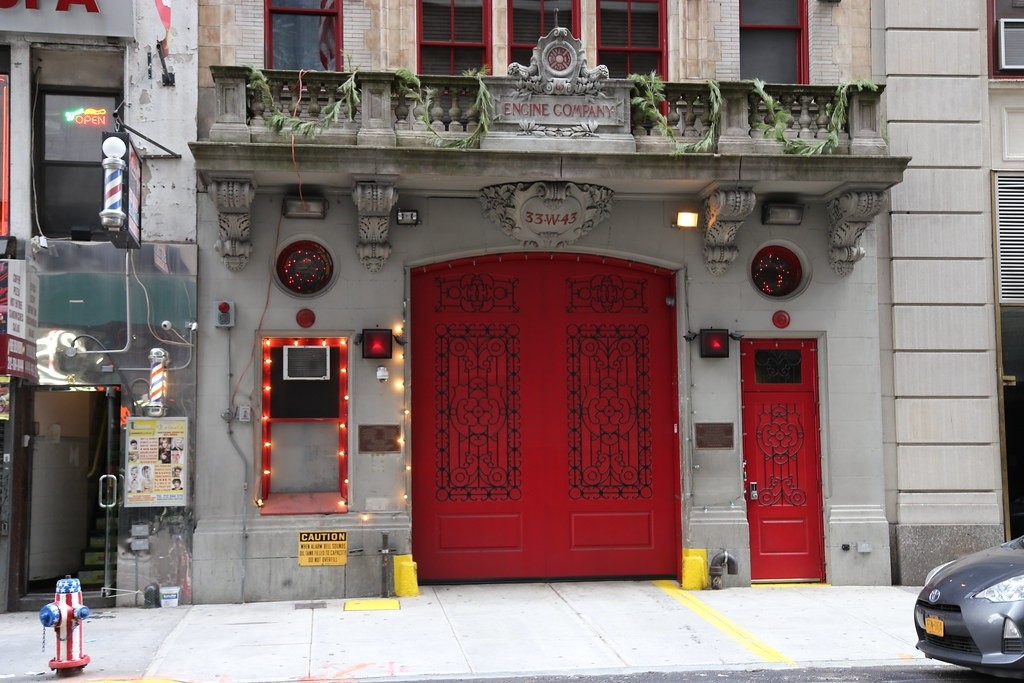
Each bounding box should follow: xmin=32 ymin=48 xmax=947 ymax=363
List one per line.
xmin=128 ymin=466 xmax=153 ymax=493
xmin=159 ymin=437 xmax=170 ymax=463
xmin=128 ymin=439 xmax=139 ymax=461
xmin=171 ymin=451 xmax=182 ymax=464
xmin=174 ymin=467 xmax=181 ymax=476
xmin=172 ymin=479 xmax=183 ymax=490
xmin=172 ymin=438 xmax=183 ymax=450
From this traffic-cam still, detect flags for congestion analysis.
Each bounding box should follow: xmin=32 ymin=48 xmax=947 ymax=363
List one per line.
xmin=318 ymin=0 xmax=336 ymax=70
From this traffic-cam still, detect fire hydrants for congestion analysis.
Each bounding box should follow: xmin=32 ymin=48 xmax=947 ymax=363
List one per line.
xmin=40 ymin=575 xmax=89 ymax=677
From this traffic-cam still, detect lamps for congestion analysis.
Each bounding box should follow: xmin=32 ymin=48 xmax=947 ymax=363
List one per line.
xmin=671 ymin=212 xmax=698 ymax=229
xmin=397 ymin=209 xmax=422 ymax=227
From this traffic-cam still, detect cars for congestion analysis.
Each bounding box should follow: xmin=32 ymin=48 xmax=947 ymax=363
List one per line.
xmin=914 ymin=535 xmax=1024 ymax=679
